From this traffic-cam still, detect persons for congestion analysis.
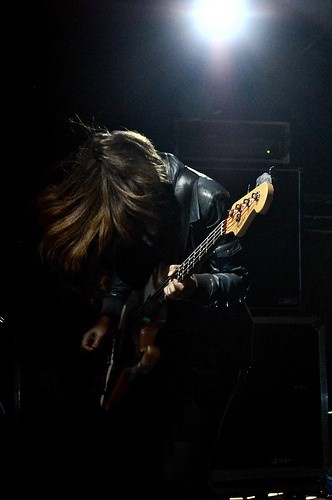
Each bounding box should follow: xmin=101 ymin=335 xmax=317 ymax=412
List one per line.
xmin=39 ymin=107 xmax=254 ymax=500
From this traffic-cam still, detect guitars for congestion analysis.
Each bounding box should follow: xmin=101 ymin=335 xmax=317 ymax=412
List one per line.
xmin=96 ymin=181 xmax=276 ymax=410
